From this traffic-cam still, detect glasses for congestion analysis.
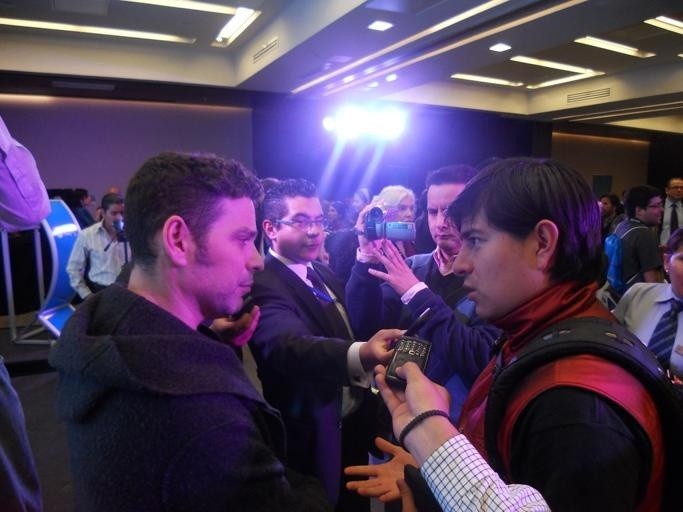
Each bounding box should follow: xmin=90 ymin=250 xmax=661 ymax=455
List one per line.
xmin=275 ymin=218 xmax=328 ymax=231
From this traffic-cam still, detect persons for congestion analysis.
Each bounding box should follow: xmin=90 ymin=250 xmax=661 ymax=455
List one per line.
xmin=52 ymin=148 xmax=683 ymax=511
xmin=0 ymin=117 xmax=52 ymax=511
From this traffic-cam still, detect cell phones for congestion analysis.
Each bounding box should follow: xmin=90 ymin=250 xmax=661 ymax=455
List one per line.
xmin=386 ymin=336 xmax=432 ymax=389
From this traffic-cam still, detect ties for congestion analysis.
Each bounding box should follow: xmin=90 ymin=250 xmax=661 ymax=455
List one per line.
xmin=307 ymin=268 xmax=352 ymax=340
xmin=670 ymin=204 xmax=678 ymax=234
xmin=648 ymin=300 xmax=682 ymax=372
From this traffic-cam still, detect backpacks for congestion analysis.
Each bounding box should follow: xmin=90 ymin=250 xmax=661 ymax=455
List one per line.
xmin=604 ymin=222 xmax=649 ymax=292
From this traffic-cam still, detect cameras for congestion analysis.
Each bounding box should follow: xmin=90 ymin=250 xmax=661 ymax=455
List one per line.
xmin=362 ymin=208 xmax=417 ymax=242
